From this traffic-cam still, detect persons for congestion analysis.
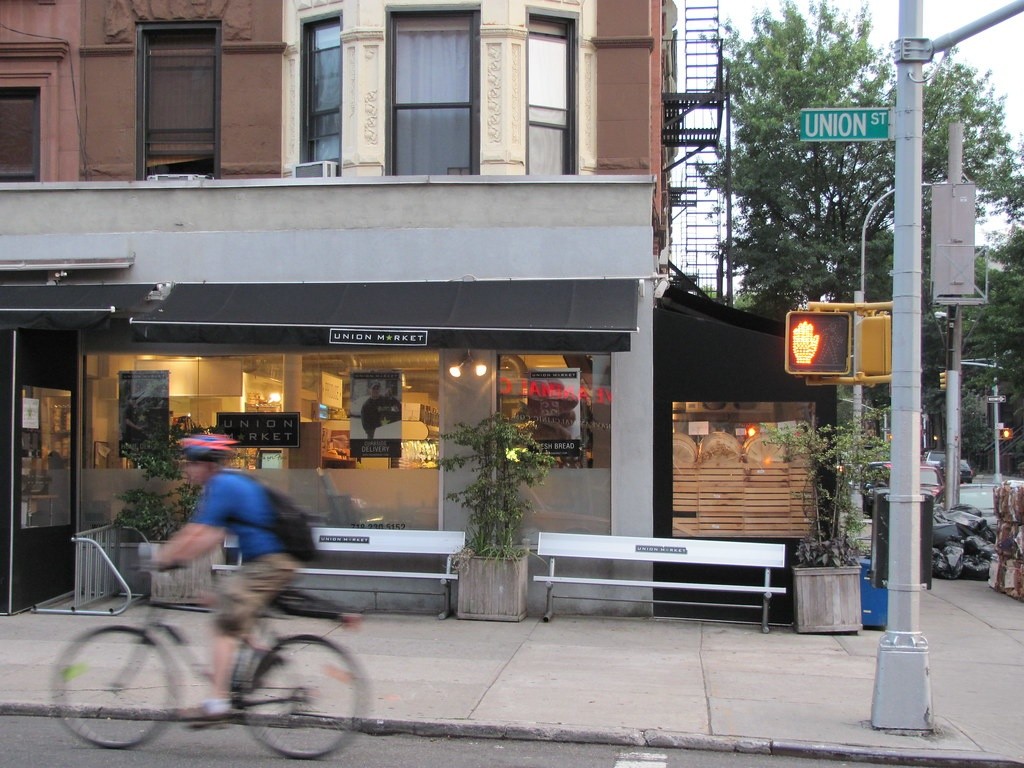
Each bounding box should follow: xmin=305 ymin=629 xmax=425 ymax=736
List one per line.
xmin=39 ymin=451 xmax=71 ymax=525
xmin=154 ymin=434 xmax=304 ymax=731
xmin=361 ymin=380 xmax=402 ymax=439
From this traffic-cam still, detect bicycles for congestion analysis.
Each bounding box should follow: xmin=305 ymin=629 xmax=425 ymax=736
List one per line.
xmin=49 ymin=561 xmax=373 ymax=764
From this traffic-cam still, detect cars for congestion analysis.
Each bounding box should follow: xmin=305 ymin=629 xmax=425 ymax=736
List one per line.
xmin=861 ymin=461 xmax=891 ymax=506
xmin=920 ymin=463 xmax=945 ymax=503
xmin=959 ymin=460 xmax=974 ymax=485
xmin=960 ymin=482 xmax=999 ymax=525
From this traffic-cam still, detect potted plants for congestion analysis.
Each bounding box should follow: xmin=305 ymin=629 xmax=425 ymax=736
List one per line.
xmin=428 ymin=411 xmax=558 ymax=621
xmin=758 ymin=405 xmax=890 ymax=634
xmin=112 ymin=415 xmax=232 ymax=604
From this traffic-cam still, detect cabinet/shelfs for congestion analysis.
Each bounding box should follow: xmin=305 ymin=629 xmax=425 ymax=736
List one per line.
xmin=222 ymin=393 xmax=440 ymax=470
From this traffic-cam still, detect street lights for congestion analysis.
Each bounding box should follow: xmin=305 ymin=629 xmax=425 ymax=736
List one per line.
xmin=933 ymin=310 xmax=1000 ymax=483
xmin=852 ymin=181 xmax=948 ymax=441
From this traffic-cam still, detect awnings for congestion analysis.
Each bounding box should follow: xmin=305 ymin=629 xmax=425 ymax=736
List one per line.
xmin=0 ymin=282 xmax=155 ymax=331
xmin=129 ymin=279 xmax=641 ymax=357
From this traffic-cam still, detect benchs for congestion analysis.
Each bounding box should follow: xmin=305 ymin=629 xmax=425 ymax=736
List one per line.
xmin=212 ymin=527 xmax=465 ymax=619
xmin=532 ymin=531 xmax=786 ymax=634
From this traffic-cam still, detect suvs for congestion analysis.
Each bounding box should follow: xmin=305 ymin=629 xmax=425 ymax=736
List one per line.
xmin=923 ymin=450 xmax=946 ymax=468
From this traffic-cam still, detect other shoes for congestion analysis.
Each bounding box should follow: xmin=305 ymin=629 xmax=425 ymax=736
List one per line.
xmin=185 ymin=701 xmax=235 ymax=730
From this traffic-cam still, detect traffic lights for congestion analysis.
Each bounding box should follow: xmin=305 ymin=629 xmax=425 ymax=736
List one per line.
xmin=785 ymin=315 xmax=850 ymax=376
xmin=1003 ymin=428 xmax=1013 ymax=439
xmin=939 ymin=369 xmax=947 ymax=390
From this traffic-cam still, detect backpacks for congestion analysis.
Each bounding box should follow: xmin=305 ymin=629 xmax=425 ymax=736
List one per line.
xmin=217 ymin=471 xmax=319 ymax=561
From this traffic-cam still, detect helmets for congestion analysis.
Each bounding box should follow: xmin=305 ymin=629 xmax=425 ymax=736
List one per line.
xmin=175 ymin=430 xmax=239 ymax=464
